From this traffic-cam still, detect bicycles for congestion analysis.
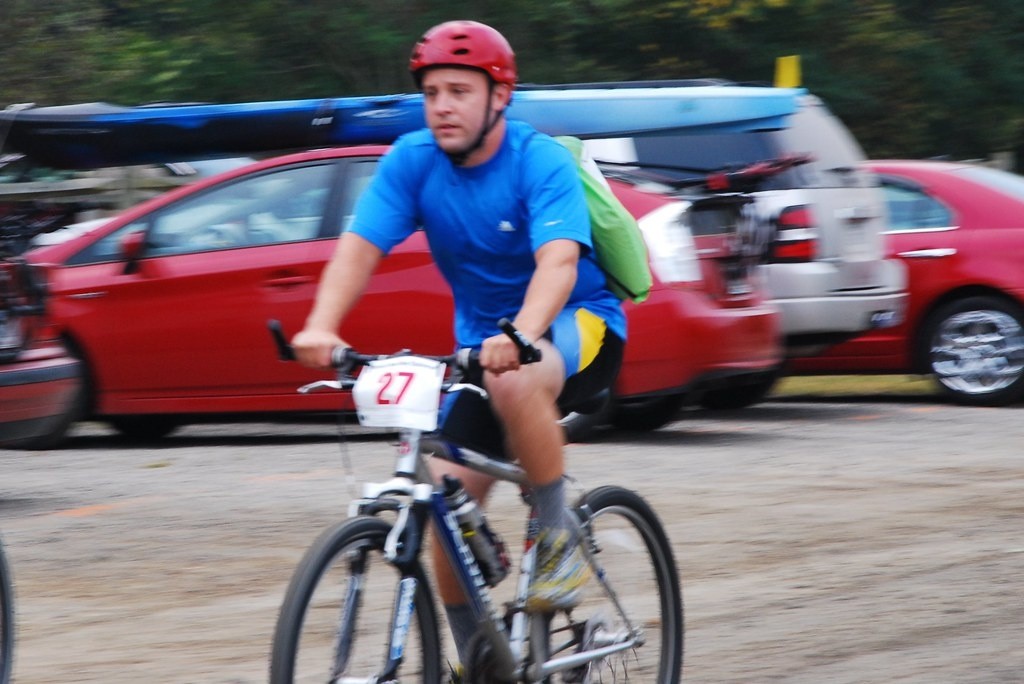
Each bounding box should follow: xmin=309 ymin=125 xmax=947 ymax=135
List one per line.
xmin=261 ymin=317 xmax=688 ymax=684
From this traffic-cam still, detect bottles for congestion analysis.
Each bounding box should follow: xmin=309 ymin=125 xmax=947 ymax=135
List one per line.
xmin=442 ymin=473 xmax=509 ymax=587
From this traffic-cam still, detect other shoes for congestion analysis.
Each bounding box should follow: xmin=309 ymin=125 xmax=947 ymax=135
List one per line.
xmin=526 ymin=526 xmax=591 ymax=609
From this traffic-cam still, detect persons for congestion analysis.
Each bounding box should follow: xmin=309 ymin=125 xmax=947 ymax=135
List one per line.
xmin=290 ymin=20 xmax=628 ymax=684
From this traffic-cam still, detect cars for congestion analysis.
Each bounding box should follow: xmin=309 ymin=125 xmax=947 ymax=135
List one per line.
xmin=0 ymin=149 xmax=827 ymax=453
xmin=31 ymin=77 xmax=912 ymax=413
xmin=767 ymin=163 xmax=1024 ymax=407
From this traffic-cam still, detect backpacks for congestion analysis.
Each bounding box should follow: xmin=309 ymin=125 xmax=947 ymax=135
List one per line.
xmin=416 ymin=135 xmax=653 ymax=304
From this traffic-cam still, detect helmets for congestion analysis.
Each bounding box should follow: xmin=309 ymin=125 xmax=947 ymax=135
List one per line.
xmin=411 ymin=21 xmax=517 ymax=86
